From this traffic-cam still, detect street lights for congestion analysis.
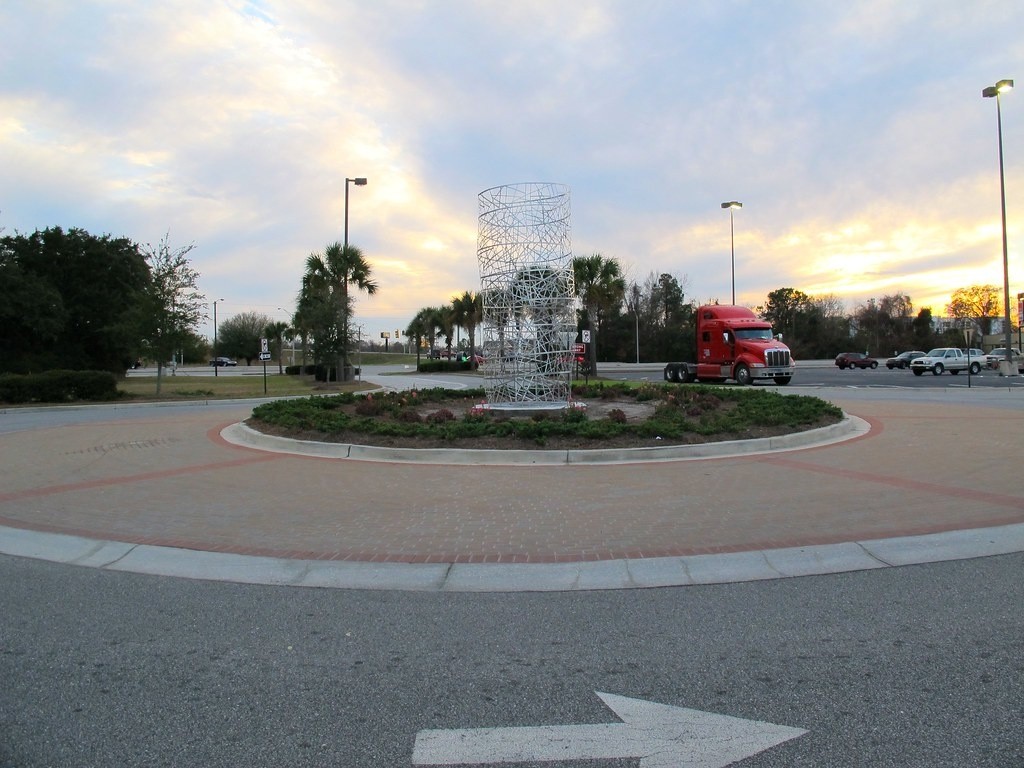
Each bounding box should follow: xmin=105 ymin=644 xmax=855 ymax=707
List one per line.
xmin=341 ymin=177 xmax=369 ymax=380
xmin=277 ymin=307 xmax=296 ymax=366
xmin=720 ymin=200 xmax=743 ymax=305
xmin=213 ymin=298 xmax=224 ymax=377
xmin=982 ymin=78 xmax=1013 ymax=362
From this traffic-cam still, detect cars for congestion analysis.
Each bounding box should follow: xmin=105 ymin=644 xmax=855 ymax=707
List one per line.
xmin=982 ymin=348 xmax=1022 ymax=369
xmin=208 ymin=356 xmax=237 ymax=367
xmin=284 ymin=358 xmax=362 ymax=376
xmin=129 ymin=358 xmax=141 ymax=369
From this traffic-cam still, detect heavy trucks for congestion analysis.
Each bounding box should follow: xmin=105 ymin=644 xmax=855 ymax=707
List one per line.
xmin=663 ymin=304 xmax=796 ymax=386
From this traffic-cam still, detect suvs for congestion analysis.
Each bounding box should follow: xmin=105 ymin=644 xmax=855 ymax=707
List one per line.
xmin=886 ymin=351 xmax=926 ymax=369
xmin=835 ymin=353 xmax=879 ymax=370
xmin=440 ymin=349 xmax=456 ymax=358
xmin=427 ymin=349 xmax=441 ymax=360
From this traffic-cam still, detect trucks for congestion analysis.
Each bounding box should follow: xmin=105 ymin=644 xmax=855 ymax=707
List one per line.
xmin=910 ymin=347 xmax=988 ymax=376
